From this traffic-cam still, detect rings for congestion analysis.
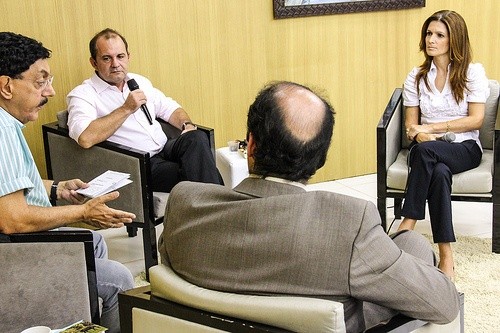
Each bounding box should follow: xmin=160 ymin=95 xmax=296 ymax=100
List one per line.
xmin=406 ymin=129 xmax=409 ymax=131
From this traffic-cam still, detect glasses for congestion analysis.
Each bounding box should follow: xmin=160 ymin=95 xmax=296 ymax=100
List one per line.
xmin=25 ymin=75 xmax=54 ymax=90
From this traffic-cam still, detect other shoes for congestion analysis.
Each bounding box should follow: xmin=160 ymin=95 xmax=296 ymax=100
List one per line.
xmin=437 ymin=260 xmax=455 ymax=284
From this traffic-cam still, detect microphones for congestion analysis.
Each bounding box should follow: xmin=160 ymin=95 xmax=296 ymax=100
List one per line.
xmin=127 ymin=78 xmax=154 ymax=125
xmin=436 ymin=131 xmax=456 ymax=143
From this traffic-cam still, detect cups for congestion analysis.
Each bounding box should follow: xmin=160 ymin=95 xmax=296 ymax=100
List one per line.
xmin=228 ymin=141 xmax=240 ymax=154
xmin=20 ymin=326 xmax=52 ymax=333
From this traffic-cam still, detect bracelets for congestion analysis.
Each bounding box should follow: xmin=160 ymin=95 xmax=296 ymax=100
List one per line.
xmin=446 ymin=121 xmax=451 ymax=133
xmin=182 ymin=122 xmax=197 ymax=130
xmin=50 ymin=180 xmax=61 ymax=201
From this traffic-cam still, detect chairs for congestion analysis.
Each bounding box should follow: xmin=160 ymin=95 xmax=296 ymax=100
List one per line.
xmin=42 ymin=110 xmax=214 ymax=283
xmin=377 ymin=81 xmax=500 ymax=254
xmin=0 ymin=230 xmax=102 ymax=333
xmin=117 ymin=264 xmax=466 ymax=333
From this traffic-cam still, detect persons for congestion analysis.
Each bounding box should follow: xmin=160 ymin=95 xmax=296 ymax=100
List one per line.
xmin=158 ymin=82 xmax=460 ymax=333
xmin=396 ymin=10 xmax=490 ymax=283
xmin=66 ymin=28 xmax=225 ymax=193
xmin=0 ymin=30 xmax=136 ymax=333
